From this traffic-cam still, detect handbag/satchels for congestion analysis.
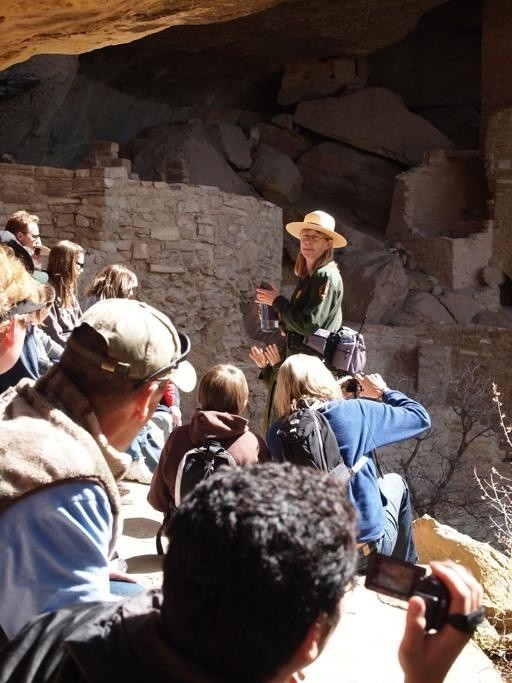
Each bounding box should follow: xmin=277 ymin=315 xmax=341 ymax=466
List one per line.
xmin=300 ymin=322 xmax=368 ymax=374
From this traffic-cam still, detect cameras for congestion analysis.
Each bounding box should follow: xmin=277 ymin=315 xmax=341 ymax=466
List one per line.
xmin=366 ymin=553 xmax=451 ymax=632
xmin=346 ymin=380 xmax=362 ymax=393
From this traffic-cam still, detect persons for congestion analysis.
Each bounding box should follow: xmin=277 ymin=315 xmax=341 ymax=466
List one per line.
xmin=247 ymin=342 xmax=287 ymax=435
xmin=0 ymin=210 xmax=199 ymax=486
xmin=253 ymin=210 xmax=348 ymax=366
xmin=145 ymin=363 xmax=274 ymax=537
xmin=265 ymin=351 xmax=434 ymax=579
xmin=0 ymin=293 xmax=194 ymax=643
xmin=0 ymin=459 xmax=486 ymax=683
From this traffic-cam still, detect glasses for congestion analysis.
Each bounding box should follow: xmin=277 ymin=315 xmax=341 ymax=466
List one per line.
xmin=76 ymin=261 xmax=84 ymax=268
xmin=133 ymin=332 xmax=191 ymax=389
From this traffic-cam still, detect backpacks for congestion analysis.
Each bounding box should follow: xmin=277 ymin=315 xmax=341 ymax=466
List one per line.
xmin=277 ymin=396 xmax=368 ymax=490
xmin=167 ymin=440 xmax=236 ymax=510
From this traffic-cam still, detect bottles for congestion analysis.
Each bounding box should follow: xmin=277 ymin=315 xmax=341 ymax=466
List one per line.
xmin=260 ymin=280 xmax=279 ymax=332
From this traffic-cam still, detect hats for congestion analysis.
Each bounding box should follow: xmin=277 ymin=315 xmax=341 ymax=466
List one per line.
xmin=285 ymin=210 xmax=347 ymax=249
xmin=0 ymin=299 xmax=41 ymax=322
xmin=66 ymin=299 xmax=197 ymax=392
xmin=33 ymin=236 xmax=50 ymax=256
xmin=33 ymin=271 xmax=49 ymax=284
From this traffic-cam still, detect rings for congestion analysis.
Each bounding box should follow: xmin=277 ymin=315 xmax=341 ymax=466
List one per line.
xmin=260 ymin=297 xmax=263 ymax=301
